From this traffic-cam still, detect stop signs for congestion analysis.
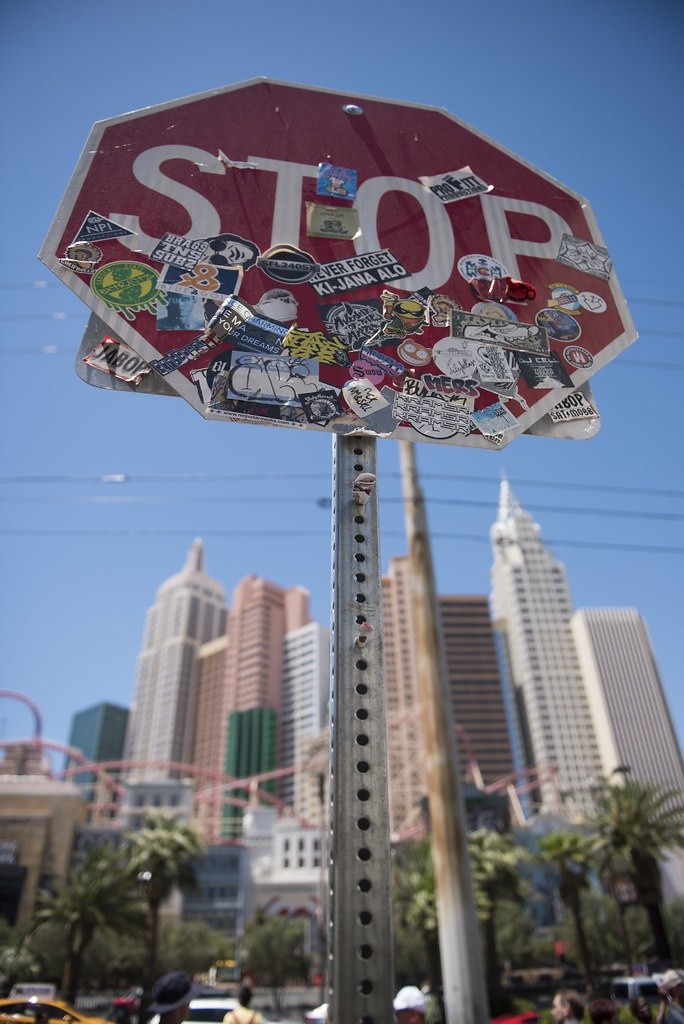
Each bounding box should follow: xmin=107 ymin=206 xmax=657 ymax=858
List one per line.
xmin=38 ymin=76 xmax=638 ymax=451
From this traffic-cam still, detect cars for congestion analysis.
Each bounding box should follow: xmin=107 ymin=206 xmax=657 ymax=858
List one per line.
xmin=0 ymin=996 xmax=115 ymax=1024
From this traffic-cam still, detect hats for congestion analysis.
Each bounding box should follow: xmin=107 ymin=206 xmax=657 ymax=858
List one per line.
xmin=141 ymin=974 xmax=210 ymax=1012
xmin=393 ymin=985 xmax=428 ymax=1012
xmin=652 ymin=969 xmax=684 ymax=994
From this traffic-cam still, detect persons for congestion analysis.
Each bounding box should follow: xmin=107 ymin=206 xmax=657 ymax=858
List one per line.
xmin=147 ymin=970 xmax=203 ymax=1024
xmin=222 ymin=987 xmax=264 ymax=1024
xmin=392 ymin=984 xmax=426 ymax=1024
xmin=630 ymin=955 xmax=684 ymax=1024
xmin=549 ymin=987 xmax=586 ymax=1024
xmin=588 ymin=998 xmax=621 ymax=1024
